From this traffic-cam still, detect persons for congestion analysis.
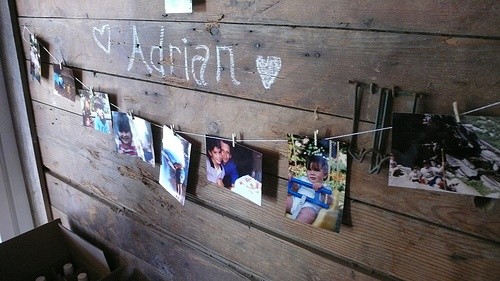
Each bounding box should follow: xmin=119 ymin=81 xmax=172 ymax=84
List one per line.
xmin=34 ymin=52 xmax=40 ymax=75
xmin=427 ymin=158 xmax=451 ymax=191
xmin=30 ymin=47 xmax=35 ymax=73
xmin=390 ymin=153 xmax=402 ymax=178
xmin=83 ymin=93 xmax=93 ymax=128
xmin=115 ymin=113 xmax=138 ymax=156
xmin=206 ymin=137 xmax=226 ymax=188
xmin=408 ymin=165 xmax=426 ymax=184
xmin=176 ymin=162 xmax=185 ymax=195
xmin=219 ymin=139 xmax=238 ymax=188
xmin=284 ymin=154 xmax=333 ymax=225
xmin=94 ymin=103 xmax=110 ymax=134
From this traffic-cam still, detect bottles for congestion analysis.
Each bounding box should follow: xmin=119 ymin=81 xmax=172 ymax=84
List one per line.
xmin=35 ymin=276 xmax=46 ymax=281
xmin=62 ymin=263 xmax=77 ymax=281
xmin=78 ymin=272 xmax=89 ymax=281
